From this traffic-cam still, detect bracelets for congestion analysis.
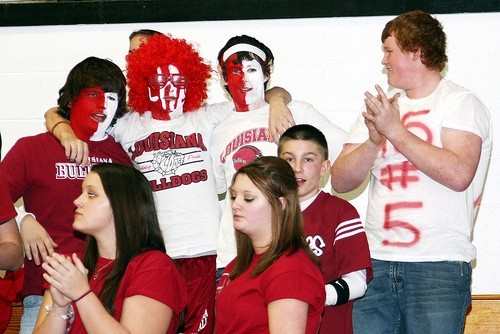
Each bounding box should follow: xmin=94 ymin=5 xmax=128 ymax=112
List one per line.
xmin=45 ymin=303 xmax=75 ymax=334
xmin=51 ymin=121 xmax=70 ymax=135
xmin=74 ymin=289 xmax=92 ymax=303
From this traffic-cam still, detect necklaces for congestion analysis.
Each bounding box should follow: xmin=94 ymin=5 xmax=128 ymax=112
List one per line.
xmin=93 ymin=258 xmax=116 ymax=280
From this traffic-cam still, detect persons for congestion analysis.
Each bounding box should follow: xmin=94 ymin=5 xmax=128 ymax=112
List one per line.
xmin=32 ymin=163 xmax=188 ymax=334
xmin=120 ymin=29 xmax=162 ymax=102
xmin=44 ymin=33 xmax=296 ymax=334
xmin=0 ymin=57 xmax=134 ymax=334
xmin=214 ymin=157 xmax=326 ymax=334
xmin=210 ymin=35 xmax=350 ymax=291
xmin=277 ymin=124 xmax=374 ymax=334
xmin=332 ymin=9 xmax=493 ymax=334
